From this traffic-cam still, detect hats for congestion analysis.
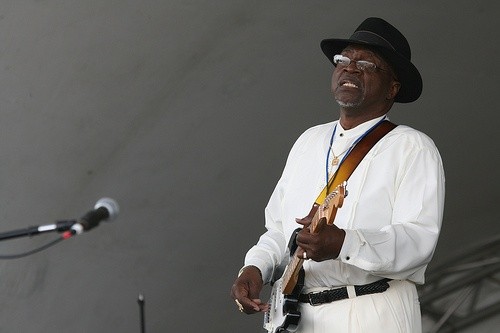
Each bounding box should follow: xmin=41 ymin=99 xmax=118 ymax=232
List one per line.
xmin=320 ymin=17 xmax=423 ymax=103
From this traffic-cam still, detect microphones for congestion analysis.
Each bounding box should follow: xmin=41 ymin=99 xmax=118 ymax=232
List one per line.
xmin=70 ymin=197 xmax=119 ymax=235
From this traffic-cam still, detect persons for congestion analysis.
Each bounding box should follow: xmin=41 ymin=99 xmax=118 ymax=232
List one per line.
xmin=231 ymin=16 xmax=445 ymax=333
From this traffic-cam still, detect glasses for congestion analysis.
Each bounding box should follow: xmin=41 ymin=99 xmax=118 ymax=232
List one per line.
xmin=333 ymin=55 xmax=397 ymax=82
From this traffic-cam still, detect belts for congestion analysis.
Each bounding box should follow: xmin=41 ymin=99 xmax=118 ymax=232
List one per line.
xmin=298 ymin=278 xmax=393 ymax=306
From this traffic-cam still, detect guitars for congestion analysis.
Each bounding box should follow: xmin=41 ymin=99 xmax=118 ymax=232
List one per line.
xmin=262 ymin=180 xmax=350 ymax=333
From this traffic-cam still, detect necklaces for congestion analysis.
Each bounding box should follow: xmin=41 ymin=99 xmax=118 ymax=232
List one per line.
xmin=330 ymin=146 xmax=348 ymax=165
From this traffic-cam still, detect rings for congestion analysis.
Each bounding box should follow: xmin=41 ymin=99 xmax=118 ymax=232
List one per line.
xmin=235 ymin=298 xmax=244 ymax=313
xmin=303 ymin=251 xmax=307 ymax=260
